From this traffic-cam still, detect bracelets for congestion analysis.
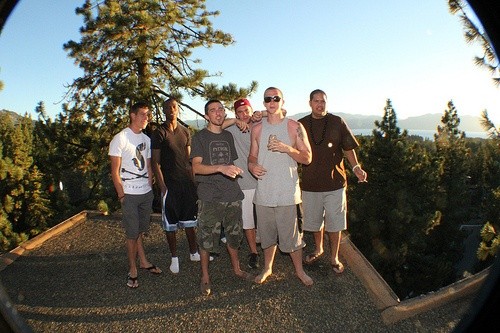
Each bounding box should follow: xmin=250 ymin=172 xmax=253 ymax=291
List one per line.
xmin=352 ymin=165 xmax=360 ymax=172
xmin=118 ymin=195 xmax=125 ymax=201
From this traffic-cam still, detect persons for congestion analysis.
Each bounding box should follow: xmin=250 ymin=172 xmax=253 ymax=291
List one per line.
xmin=248 ymin=87 xmax=313 ymax=285
xmin=297 ymin=89 xmax=367 ymax=273
xmin=221 ymin=99 xmax=306 ymax=268
xmin=151 ymin=98 xmax=213 ymax=274
xmin=108 ymin=103 xmax=162 ymax=288
xmin=189 ymin=100 xmax=253 ymax=295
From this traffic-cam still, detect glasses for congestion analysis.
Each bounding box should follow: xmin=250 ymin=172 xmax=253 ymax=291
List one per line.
xmin=264 ymin=96 xmax=282 ymax=103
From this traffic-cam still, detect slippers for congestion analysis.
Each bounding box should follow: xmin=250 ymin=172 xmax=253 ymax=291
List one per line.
xmin=139 ymin=264 xmax=162 ymax=274
xmin=330 ymin=260 xmax=344 ymax=274
xmin=127 ymin=273 xmax=139 ymax=287
xmin=303 ymin=251 xmax=324 ymax=265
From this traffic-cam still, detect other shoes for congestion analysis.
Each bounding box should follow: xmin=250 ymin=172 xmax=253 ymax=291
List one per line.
xmin=169 ymin=256 xmax=179 ymax=274
xmin=220 ymin=237 xmax=227 ymax=243
xmin=190 ymin=252 xmax=214 ymax=262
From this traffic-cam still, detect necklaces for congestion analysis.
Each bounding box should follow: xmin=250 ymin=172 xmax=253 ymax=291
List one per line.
xmin=310 ymin=113 xmax=328 ymax=145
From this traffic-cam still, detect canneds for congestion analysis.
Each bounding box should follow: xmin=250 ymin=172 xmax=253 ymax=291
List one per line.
xmin=269 ymin=134 xmax=277 ymax=144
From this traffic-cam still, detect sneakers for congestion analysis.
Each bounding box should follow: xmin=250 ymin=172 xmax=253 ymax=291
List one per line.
xmin=247 ymin=253 xmax=258 ymax=268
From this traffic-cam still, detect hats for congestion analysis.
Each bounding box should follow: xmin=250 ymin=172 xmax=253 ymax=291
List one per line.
xmin=234 ymin=99 xmax=250 ymax=112
